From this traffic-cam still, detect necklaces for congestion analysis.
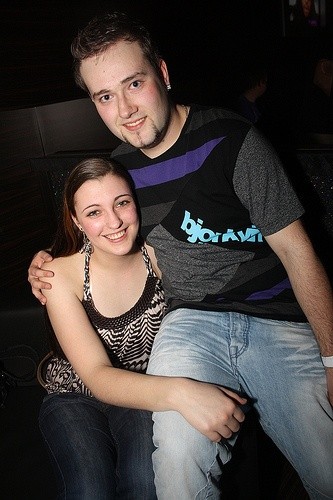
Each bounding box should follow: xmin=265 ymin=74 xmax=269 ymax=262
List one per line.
xmin=182 ymin=105 xmax=189 ymax=119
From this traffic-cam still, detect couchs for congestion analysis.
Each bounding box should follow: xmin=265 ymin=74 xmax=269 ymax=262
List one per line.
xmin=0 ymin=294 xmax=263 ymax=500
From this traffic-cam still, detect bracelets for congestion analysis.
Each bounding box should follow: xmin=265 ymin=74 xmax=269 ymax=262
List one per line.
xmin=319 ymin=354 xmax=333 ymax=368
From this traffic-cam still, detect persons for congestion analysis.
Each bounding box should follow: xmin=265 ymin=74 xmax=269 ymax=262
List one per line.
xmin=28 ymin=10 xmax=333 ymax=500
xmin=33 ymin=158 xmax=247 ymax=500
